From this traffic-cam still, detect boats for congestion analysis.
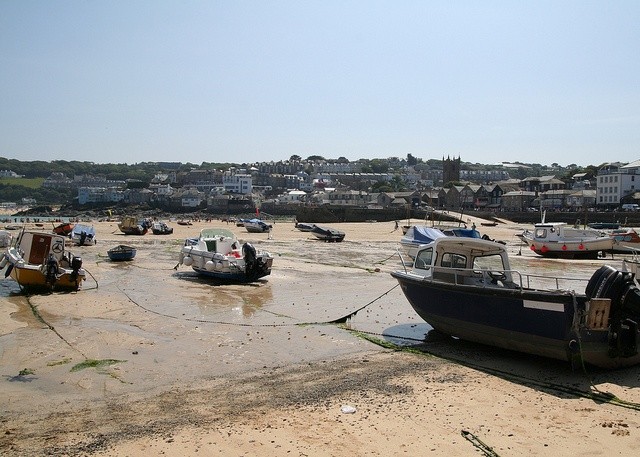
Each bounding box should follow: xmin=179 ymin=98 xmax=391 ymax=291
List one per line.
xmin=390 ymin=236 xmax=639 ymax=372
xmin=310 ymin=224 xmax=346 ymax=242
xmin=399 ymin=225 xmax=508 ymax=263
xmin=245 ymin=218 xmax=273 ymax=233
xmin=514 ymin=197 xmax=616 ymax=256
xmin=152 ymin=220 xmax=174 ymax=234
xmin=107 ymin=244 xmax=136 ymax=261
xmin=182 ymin=228 xmax=273 ymax=281
xmin=143 ymin=217 xmax=154 ymax=227
xmin=295 ymin=223 xmax=315 ymax=232
xmin=236 ymin=218 xmax=245 ymax=226
xmin=68 ymin=223 xmax=97 ymax=245
xmin=177 ymin=216 xmax=193 ymax=225
xmin=589 ymin=222 xmax=620 ymax=229
xmin=45 ymin=211 xmax=74 ymax=236
xmin=601 ymin=227 xmax=640 ymax=254
xmin=4 ymin=228 xmax=89 ymax=290
xmin=117 ymin=214 xmax=146 ymax=235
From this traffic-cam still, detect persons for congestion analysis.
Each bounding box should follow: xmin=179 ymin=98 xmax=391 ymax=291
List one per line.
xmin=43 ymin=253 xmax=59 ymax=292
xmin=471 ymin=222 xmax=476 ymax=230
xmin=463 ymin=224 xmax=467 ymax=229
